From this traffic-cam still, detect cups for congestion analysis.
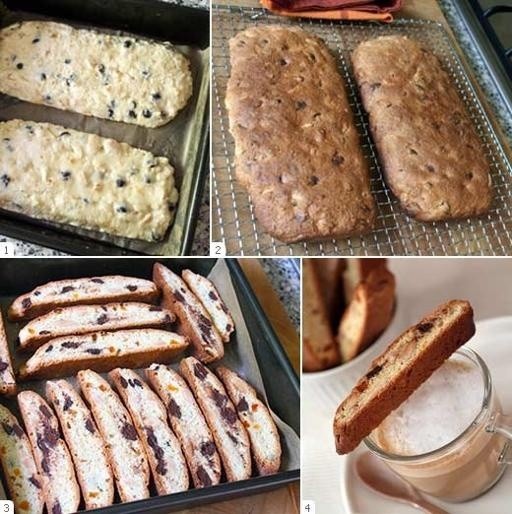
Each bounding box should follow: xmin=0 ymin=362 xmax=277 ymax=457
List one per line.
xmin=363 ymin=345 xmax=512 ymax=503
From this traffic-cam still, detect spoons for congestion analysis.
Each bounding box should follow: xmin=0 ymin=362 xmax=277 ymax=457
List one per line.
xmin=353 ymin=454 xmax=449 ymax=513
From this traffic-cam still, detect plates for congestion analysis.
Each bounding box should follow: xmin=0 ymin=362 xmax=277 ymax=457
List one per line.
xmin=340 ymin=415 xmax=512 ymax=514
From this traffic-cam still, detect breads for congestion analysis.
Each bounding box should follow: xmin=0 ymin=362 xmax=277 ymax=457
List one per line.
xmin=0 ymin=21 xmax=192 ymax=129
xmin=333 ymin=298 xmax=475 ymax=455
xmin=227 ymin=24 xmax=374 ymax=243
xmin=0 ymin=119 xmax=179 ymax=240
xmin=302 ymin=258 xmax=396 ymax=371
xmin=0 ymin=262 xmax=282 ymax=514
xmin=350 ymin=37 xmax=492 ymax=221
